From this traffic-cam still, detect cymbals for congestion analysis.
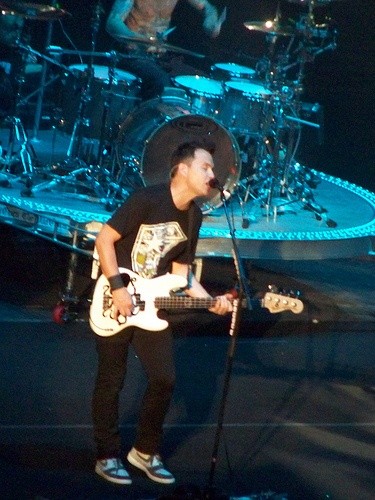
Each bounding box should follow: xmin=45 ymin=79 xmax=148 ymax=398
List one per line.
xmin=0 ymin=0 xmax=66 ymax=20
xmin=243 ymin=21 xmax=327 ymax=38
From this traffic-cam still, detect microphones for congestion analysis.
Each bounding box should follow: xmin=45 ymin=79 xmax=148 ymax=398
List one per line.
xmin=208 ymin=177 xmax=230 ymax=196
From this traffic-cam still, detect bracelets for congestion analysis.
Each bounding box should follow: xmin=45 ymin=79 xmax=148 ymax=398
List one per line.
xmin=108 ymin=274 xmax=123 ymax=290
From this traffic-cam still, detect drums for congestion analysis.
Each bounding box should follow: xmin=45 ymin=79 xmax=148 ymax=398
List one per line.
xmin=59 ymin=64 xmax=143 ymax=139
xmin=220 ymin=80 xmax=274 ymax=137
xmin=116 ymin=94 xmax=242 ymax=215
xmin=171 ymin=73 xmax=225 ymax=119
xmin=210 ymin=62 xmax=256 ymax=81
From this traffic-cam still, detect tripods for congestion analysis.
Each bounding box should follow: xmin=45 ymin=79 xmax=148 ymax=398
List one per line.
xmin=0 ymin=0 xmax=336 ymax=229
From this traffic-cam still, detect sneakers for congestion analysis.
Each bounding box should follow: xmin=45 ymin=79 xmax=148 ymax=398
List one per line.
xmin=127 ymin=447 xmax=174 ymax=483
xmin=95 ymin=458 xmax=133 ymax=485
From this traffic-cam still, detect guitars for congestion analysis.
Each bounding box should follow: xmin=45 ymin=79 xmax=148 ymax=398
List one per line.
xmin=92 ymin=268 xmax=304 ymax=337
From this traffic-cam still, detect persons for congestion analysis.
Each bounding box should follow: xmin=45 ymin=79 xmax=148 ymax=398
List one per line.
xmin=106 ymin=0 xmax=219 ymax=96
xmin=93 ymin=140 xmax=233 ymax=484
